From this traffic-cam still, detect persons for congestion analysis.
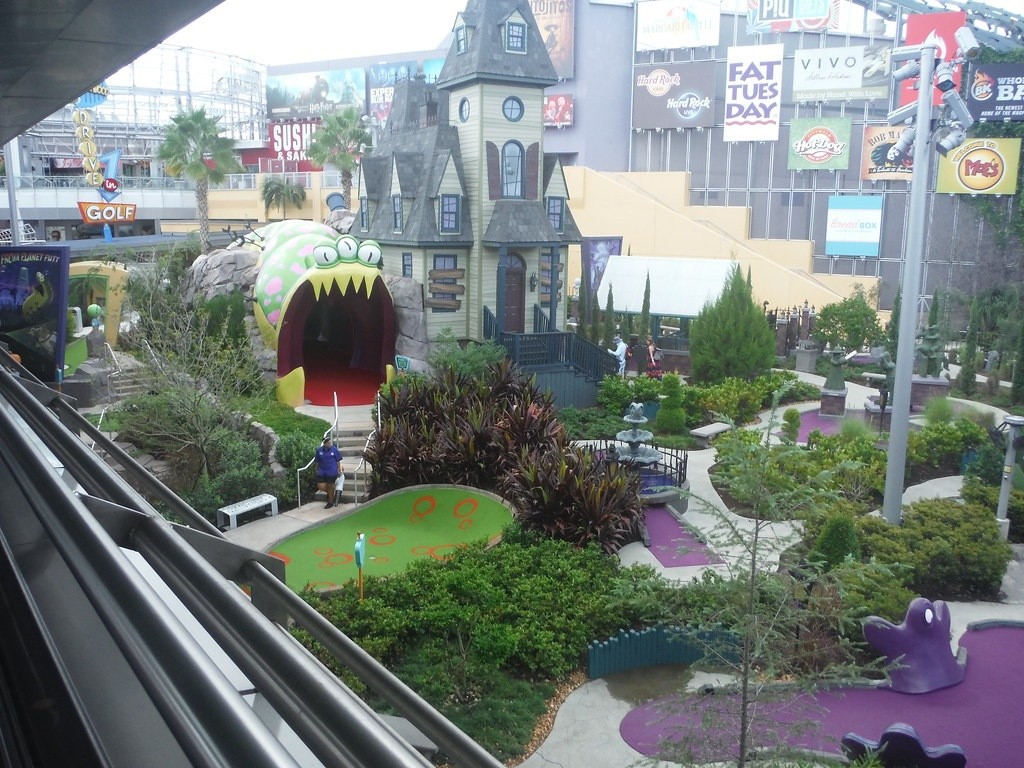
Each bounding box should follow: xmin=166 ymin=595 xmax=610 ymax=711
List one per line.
xmin=607 ymin=336 xmax=628 ymax=380
xmin=646 ymin=340 xmax=662 ymax=380
xmin=315 ymin=436 xmax=346 ymax=509
xmin=879 ymin=351 xmax=897 ymax=405
xmin=544 ymin=97 xmax=571 ymax=123
xmin=823 ymin=346 xmax=848 ymax=389
xmin=926 ymin=324 xmax=943 ymax=359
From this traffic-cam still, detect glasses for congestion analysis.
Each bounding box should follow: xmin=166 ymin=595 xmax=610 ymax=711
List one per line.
xmin=53 ymin=236 xmax=59 ymax=238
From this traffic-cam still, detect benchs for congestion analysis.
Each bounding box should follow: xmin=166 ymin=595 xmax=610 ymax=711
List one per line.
xmin=861 ymin=372 xmax=887 ymax=387
xmin=217 ymin=493 xmax=278 ymax=529
xmin=659 ymin=325 xmax=680 ymax=335
xmin=690 ymin=422 xmax=732 ymax=447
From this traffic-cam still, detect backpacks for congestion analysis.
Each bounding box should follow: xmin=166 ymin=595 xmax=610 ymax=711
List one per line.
xmin=625 ymin=343 xmax=633 ymax=359
xmin=653 ymin=346 xmax=664 ymax=360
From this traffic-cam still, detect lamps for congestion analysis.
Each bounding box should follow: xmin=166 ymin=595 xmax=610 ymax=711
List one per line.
xmin=530 ymin=272 xmax=538 ymax=292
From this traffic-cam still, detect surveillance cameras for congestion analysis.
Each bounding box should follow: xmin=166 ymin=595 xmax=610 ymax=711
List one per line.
xmin=941 ymin=89 xmax=974 ymax=129
xmin=954 ymin=27 xmax=981 ymax=60
xmin=887 ymin=100 xmax=918 ymax=126
xmin=891 ymin=44 xmax=921 ymax=62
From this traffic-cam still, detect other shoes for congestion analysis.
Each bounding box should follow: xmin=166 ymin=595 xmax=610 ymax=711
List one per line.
xmin=324 ymin=502 xmax=333 ymax=509
